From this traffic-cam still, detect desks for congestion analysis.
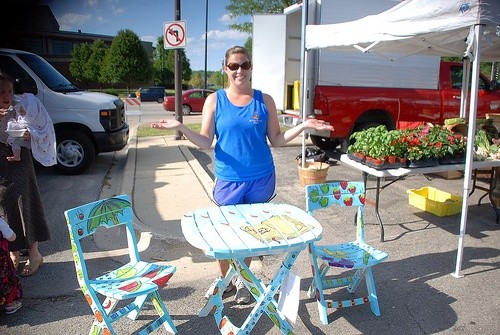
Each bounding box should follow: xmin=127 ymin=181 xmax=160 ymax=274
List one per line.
xmin=340 ymin=154 xmax=500 ymax=242
xmin=181 ymin=203 xmax=324 ymax=335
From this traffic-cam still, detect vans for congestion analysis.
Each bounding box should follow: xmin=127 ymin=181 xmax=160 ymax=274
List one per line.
xmin=1 ymin=49 xmax=129 ymax=175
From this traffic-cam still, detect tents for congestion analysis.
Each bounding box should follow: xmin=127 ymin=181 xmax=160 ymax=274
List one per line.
xmin=300 ymin=0 xmax=500 ymax=280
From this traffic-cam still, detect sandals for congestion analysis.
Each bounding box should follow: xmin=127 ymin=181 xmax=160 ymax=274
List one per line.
xmin=4 ymin=301 xmax=23 ymax=315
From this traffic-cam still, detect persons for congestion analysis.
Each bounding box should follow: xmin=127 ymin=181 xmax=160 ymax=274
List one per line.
xmin=150 ymin=46 xmax=335 ymax=305
xmin=0 ymin=214 xmax=23 ymax=315
xmin=0 ymin=74 xmax=51 ymax=277
xmin=6 ymin=93 xmax=57 ymax=166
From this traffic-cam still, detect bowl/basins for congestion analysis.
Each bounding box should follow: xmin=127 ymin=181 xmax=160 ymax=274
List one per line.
xmin=5 ymin=130 xmax=25 ymax=137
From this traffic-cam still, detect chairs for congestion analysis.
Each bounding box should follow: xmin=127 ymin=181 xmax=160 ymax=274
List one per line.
xmin=306 ymin=181 xmax=389 ymax=325
xmin=64 ymin=194 xmax=178 ymax=335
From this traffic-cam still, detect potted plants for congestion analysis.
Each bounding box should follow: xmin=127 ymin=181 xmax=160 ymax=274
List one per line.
xmin=346 ymin=124 xmax=467 ymax=165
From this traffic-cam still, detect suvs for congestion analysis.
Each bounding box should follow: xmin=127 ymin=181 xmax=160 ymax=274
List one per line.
xmin=128 ymin=86 xmax=165 ymax=104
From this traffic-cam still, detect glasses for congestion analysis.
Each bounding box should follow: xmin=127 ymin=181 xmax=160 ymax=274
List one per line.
xmin=226 ymin=61 xmax=251 ymax=71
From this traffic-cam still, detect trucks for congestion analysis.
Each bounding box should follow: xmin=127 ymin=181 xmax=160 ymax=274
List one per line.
xmin=251 ymin=0 xmax=500 ymax=152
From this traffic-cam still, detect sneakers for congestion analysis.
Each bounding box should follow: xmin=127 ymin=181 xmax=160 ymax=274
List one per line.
xmin=231 ymin=277 xmax=250 ymax=303
xmin=204 ymin=275 xmax=233 ymax=299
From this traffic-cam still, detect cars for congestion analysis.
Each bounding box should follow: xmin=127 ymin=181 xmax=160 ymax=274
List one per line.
xmin=163 ymin=88 xmax=215 ymax=116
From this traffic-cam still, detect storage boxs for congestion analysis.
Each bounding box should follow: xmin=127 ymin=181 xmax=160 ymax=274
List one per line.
xmin=406 ymin=186 xmax=462 ymax=216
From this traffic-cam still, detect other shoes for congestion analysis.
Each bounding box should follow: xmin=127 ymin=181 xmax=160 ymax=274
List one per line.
xmin=20 ymin=256 xmax=44 ymax=277
xmin=10 ymin=253 xmax=22 ymax=272
